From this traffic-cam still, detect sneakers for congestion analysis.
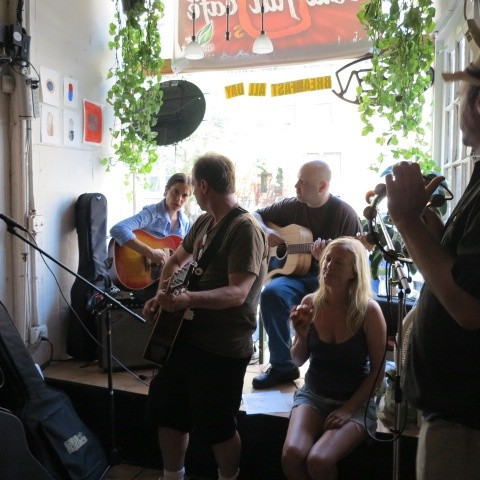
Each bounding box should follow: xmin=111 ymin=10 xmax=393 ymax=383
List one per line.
xmin=252 ymin=368 xmax=301 ymax=388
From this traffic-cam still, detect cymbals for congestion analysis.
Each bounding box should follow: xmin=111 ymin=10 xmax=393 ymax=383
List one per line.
xmin=362 ymin=171 xmax=454 ymax=265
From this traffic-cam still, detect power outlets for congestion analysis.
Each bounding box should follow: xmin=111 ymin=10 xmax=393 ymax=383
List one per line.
xmin=30 ymin=325 xmax=48 ymax=345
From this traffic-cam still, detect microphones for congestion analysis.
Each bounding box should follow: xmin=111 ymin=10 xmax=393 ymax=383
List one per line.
xmin=0 ymin=213 xmax=27 ymax=231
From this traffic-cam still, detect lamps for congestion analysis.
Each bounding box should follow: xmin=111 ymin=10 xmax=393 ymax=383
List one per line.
xmin=184 ymin=1 xmax=204 ymax=60
xmin=252 ymin=0 xmax=273 ymax=55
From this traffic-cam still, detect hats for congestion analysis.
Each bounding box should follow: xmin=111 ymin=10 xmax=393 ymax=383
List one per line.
xmin=440 ymin=51 xmax=480 ymax=86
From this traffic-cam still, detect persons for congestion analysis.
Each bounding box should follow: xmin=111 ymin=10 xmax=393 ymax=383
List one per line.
xmin=142 ymin=151 xmax=269 ymax=480
xmin=109 ymin=172 xmax=198 ymax=303
xmin=252 ymin=160 xmax=364 ymax=389
xmin=281 ymin=235 xmax=388 ymax=480
xmin=379 ymin=54 xmax=479 ymax=479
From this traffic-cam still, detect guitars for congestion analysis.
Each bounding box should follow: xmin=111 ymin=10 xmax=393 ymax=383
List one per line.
xmin=108 ymin=229 xmax=184 ymax=290
xmin=141 ymin=259 xmax=198 ymax=369
xmin=262 ymin=221 xmax=376 ymax=282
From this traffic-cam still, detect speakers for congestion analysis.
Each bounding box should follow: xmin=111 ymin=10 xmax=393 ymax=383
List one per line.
xmin=94 ymin=300 xmax=156 ymax=373
